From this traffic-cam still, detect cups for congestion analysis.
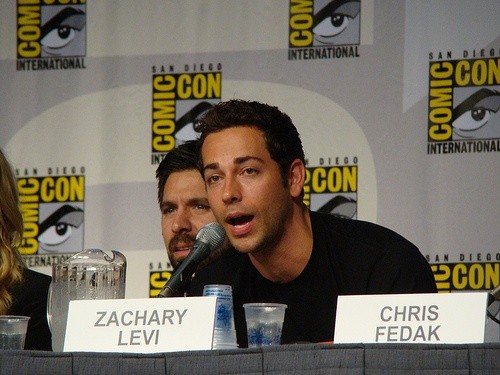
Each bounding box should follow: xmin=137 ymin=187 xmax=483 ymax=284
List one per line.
xmin=0 ymin=315 xmax=30 ymax=349
xmin=203 ymin=285 xmax=239 ymax=350
xmin=243 ymin=302 xmax=287 ymax=348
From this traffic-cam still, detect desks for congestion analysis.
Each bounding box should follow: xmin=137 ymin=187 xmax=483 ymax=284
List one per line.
xmin=0 ymin=332 xmax=500 ymax=375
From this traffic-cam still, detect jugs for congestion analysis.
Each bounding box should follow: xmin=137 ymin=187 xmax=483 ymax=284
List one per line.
xmin=46 ymin=248 xmax=127 ymax=351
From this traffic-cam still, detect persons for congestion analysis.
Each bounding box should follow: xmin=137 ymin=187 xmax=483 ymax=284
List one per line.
xmin=181 ymin=99 xmax=438 ymax=348
xmin=0 ymin=148 xmax=55 ymax=351
xmin=153 ymin=137 xmax=218 ymax=269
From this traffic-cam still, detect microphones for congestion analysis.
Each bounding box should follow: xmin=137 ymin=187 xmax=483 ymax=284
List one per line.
xmin=157 ymin=222 xmax=226 ymax=298
